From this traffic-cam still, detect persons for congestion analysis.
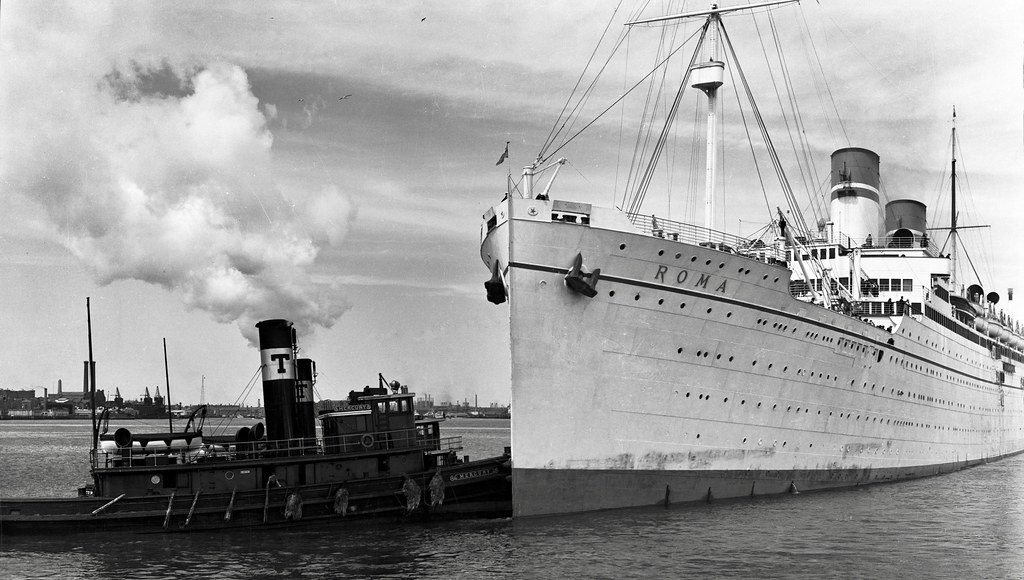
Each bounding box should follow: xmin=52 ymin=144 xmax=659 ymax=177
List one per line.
xmin=866 ymin=234 xmax=872 ymax=246
xmin=888 ymin=296 xmax=909 ymax=316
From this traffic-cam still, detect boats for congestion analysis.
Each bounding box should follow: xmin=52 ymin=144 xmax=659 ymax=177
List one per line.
xmin=476 ymin=0 xmax=1024 ymax=521
xmin=1 ymin=317 xmax=511 ymax=539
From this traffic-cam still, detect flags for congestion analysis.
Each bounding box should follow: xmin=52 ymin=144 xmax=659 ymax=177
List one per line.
xmin=496 ymin=145 xmax=509 ymax=166
